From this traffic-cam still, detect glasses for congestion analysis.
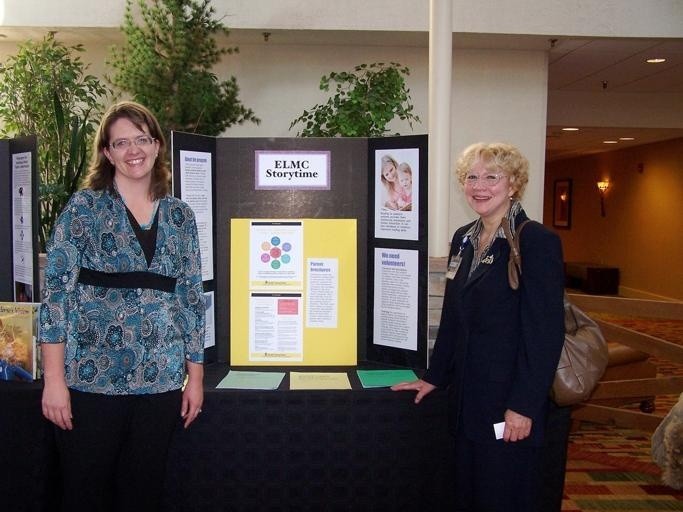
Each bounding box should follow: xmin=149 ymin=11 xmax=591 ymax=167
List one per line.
xmin=109 ymin=136 xmax=155 ymax=149
xmin=461 ymin=173 xmax=507 ymax=186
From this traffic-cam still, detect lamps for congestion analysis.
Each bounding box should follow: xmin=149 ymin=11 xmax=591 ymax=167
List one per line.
xmin=596 ymin=178 xmax=608 ymax=192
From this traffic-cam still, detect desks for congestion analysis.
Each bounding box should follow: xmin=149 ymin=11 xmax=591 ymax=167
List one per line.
xmin=0 ymin=359 xmax=571 ymax=509
xmin=562 ymin=261 xmax=621 ymax=296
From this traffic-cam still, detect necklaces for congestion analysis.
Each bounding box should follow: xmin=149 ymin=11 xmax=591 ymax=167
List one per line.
xmin=118 ymin=184 xmax=158 ymax=230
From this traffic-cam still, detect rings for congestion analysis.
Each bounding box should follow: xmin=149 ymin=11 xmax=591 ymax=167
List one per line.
xmin=197 ymin=408 xmax=202 ymax=413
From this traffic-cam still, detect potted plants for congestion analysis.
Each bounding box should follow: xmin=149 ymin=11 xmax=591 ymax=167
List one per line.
xmin=0 ymin=30 xmax=121 ymax=299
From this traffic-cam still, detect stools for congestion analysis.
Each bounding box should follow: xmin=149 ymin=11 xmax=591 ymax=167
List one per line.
xmin=567 ymin=330 xmax=658 ymax=430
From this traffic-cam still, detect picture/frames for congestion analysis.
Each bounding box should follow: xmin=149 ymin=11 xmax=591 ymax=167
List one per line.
xmin=549 ymin=175 xmax=571 ymax=230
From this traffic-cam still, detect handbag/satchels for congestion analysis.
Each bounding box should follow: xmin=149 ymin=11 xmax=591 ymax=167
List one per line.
xmin=551 ymin=297 xmax=609 ymax=407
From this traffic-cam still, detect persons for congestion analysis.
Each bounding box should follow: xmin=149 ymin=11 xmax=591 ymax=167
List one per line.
xmin=42 ymin=104 xmax=206 ymax=512
xmin=381 ymin=155 xmax=412 ymax=209
xmin=391 ymin=142 xmax=566 ymax=511
